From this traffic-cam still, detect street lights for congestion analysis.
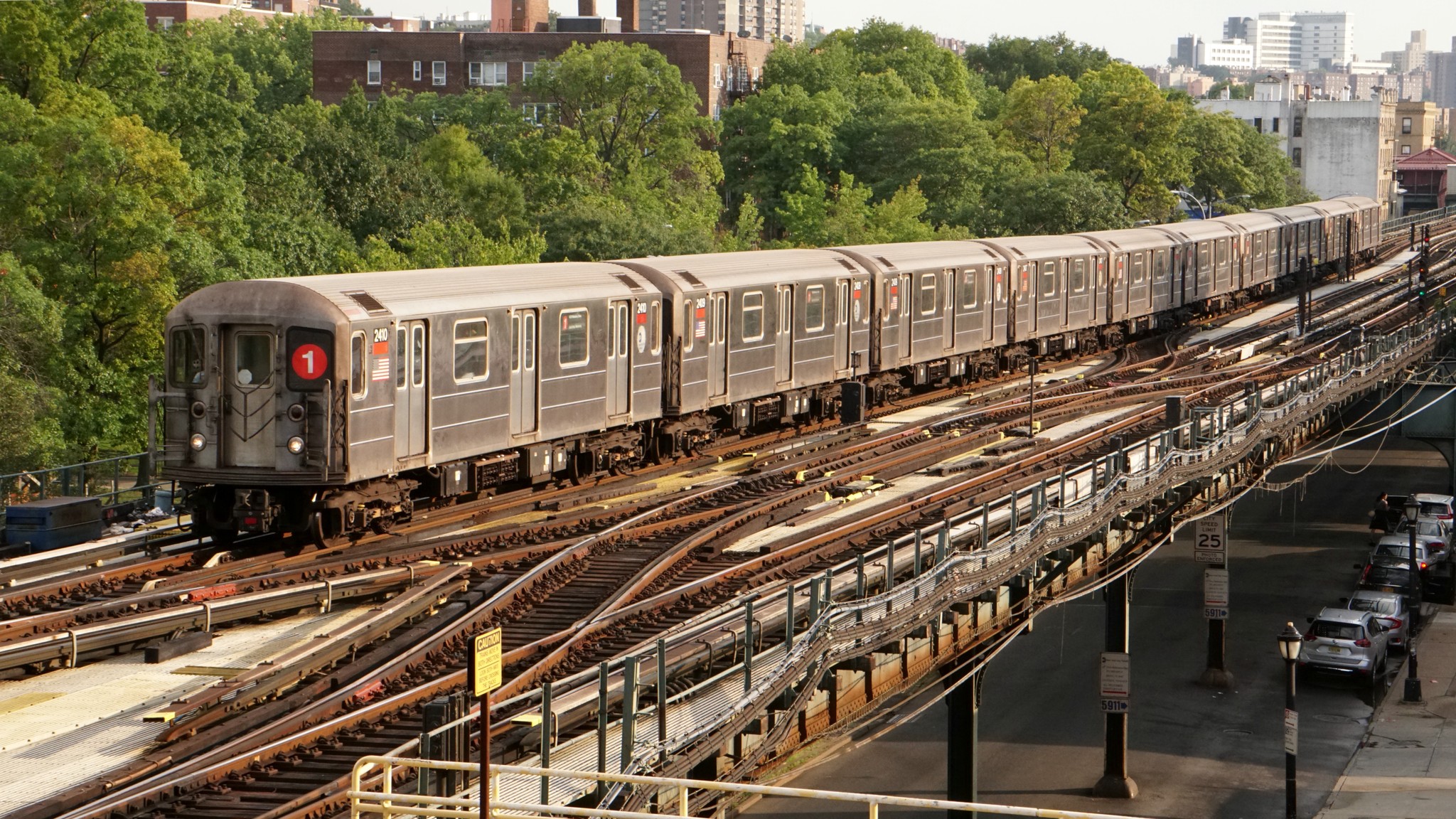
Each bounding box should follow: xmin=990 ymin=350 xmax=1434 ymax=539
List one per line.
xmin=1276 ymin=621 xmax=1304 ymax=819
xmin=1403 ymin=492 xmax=1423 ymax=638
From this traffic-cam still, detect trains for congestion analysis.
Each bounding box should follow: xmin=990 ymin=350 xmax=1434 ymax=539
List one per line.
xmin=147 ymin=192 xmax=1384 ymax=557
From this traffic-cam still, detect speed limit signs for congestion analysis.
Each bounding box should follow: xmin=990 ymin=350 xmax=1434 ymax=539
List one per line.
xmin=1194 ymin=513 xmax=1225 ymax=552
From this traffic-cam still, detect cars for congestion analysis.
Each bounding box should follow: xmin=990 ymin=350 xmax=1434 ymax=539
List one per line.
xmin=1352 ymin=554 xmax=1429 ymax=605
xmin=1392 ymin=517 xmax=1454 ymax=571
xmin=1339 ymin=590 xmax=1410 ymax=656
xmin=1368 ymin=535 xmax=1440 ymax=583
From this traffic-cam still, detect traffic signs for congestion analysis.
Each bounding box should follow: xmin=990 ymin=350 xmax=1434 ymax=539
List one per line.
xmin=1101 ymin=697 xmax=1130 ymax=714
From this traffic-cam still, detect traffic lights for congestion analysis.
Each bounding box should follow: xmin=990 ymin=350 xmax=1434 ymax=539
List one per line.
xmin=1418 ymin=257 xmax=1427 ymax=312
xmin=1424 ymin=226 xmax=1430 ymax=254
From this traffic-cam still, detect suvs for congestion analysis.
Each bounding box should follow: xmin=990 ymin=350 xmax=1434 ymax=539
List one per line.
xmin=1298 ymin=607 xmax=1391 ymax=693
xmin=1385 ymin=494 xmax=1455 ymax=537
